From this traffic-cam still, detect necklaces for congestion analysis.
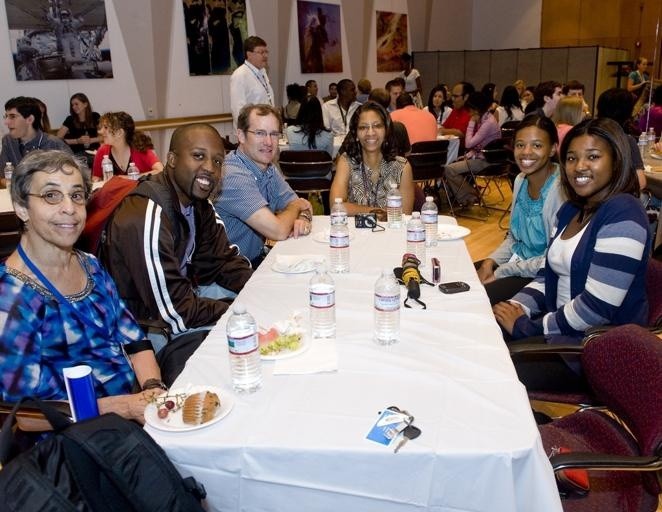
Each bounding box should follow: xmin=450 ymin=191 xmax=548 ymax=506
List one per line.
xmin=243 ymin=63 xmax=272 ymax=103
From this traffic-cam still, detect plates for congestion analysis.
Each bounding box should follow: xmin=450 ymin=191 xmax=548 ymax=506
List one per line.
xmin=144 ymin=383 xmax=237 ymax=432
xmin=259 ymin=331 xmax=310 ymax=361
xmin=436 ymin=223 xmax=469 ymax=241
xmin=314 ymin=230 xmax=354 ymax=244
xmin=649 ymin=150 xmax=661 ymax=160
xmin=273 ymin=256 xmax=322 ymax=274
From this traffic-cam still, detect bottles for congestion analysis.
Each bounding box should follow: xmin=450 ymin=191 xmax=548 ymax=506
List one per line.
xmin=307 ymin=264 xmax=337 ymax=341
xmin=373 ymin=266 xmax=401 ymax=346
xmin=329 ymin=217 xmax=351 ymax=272
xmin=128 ymin=163 xmax=140 ymax=181
xmin=639 ymin=128 xmax=656 ymax=161
xmin=407 ymin=211 xmax=427 ymax=268
xmin=85 ymin=132 xmax=90 ymax=148
xmin=282 ymin=123 xmax=288 ymax=140
xmin=102 ymin=156 xmax=114 ymax=181
xmin=227 ymin=303 xmax=264 ymax=392
xmin=386 ymin=183 xmax=403 ymax=230
xmin=422 ymin=196 xmax=439 ymax=248
xmin=330 ymin=199 xmax=349 ymax=226
xmin=5 ymin=162 xmax=16 ymax=194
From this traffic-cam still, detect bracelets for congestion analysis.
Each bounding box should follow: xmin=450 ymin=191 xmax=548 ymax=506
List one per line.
xmin=299 ymin=210 xmax=312 ymax=221
xmin=142 ymin=379 xmax=167 ymax=390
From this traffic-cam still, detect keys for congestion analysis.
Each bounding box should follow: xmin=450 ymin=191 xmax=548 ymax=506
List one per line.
xmin=393 ymin=424 xmax=420 ymax=454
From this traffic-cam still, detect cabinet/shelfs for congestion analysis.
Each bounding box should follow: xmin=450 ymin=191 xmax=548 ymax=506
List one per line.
xmin=403 ymin=44 xmax=630 ymax=129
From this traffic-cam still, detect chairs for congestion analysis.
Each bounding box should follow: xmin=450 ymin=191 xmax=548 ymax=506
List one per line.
xmin=519 ymin=321 xmax=662 ymax=512
xmin=277 ymin=149 xmax=337 ymax=217
xmin=450 ymin=136 xmax=513 ymax=221
xmin=1 ymin=394 xmax=74 ymax=434
xmin=404 ymin=137 xmax=456 ymax=217
xmin=498 ymin=159 xmax=522 ymax=230
xmin=97 ymin=220 xmax=173 ymax=348
xmin=500 ymin=120 xmax=523 ymax=144
xmin=0 ymin=211 xmax=29 ymax=264
xmin=509 ymin=258 xmax=662 ymax=408
xmin=640 ymin=190 xmax=658 ymax=258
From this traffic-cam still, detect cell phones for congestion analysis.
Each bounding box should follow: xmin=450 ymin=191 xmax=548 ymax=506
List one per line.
xmin=439 ymin=281 xmax=470 ymax=293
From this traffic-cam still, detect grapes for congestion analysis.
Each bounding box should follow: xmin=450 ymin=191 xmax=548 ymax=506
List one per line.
xmin=165 ymin=401 xmax=175 ymax=410
xmin=157 ymin=408 xmax=168 ymax=419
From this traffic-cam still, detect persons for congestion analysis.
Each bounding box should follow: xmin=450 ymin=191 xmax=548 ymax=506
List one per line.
xmin=283 ymin=79 xmax=437 ymax=215
xmin=423 ymin=79 xmax=593 ymax=211
xmin=0 ymin=91 xmax=164 ymax=187
xmin=473 ymin=113 xmax=570 ymax=308
xmin=209 ymin=102 xmax=314 ymax=269
xmin=628 ymin=56 xmax=650 ymax=104
xmin=105 ymin=123 xmax=255 ymax=385
xmin=0 ymin=146 xmax=169 ymax=446
xmin=398 ymin=52 xmax=422 ymax=97
xmin=328 ymin=101 xmax=417 ymax=222
xmin=491 ymin=117 xmax=652 ymax=396
xmin=596 ymin=88 xmax=662 ymax=209
xmin=633 ymin=84 xmax=662 ymax=143
xmin=230 ymin=35 xmax=275 ymax=139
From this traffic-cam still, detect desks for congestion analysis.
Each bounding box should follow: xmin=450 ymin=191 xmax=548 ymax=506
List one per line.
xmin=640 ymin=136 xmax=662 ymax=209
xmin=140 ymin=207 xmax=567 ymax=512
xmin=0 ymin=171 xmax=104 ymax=215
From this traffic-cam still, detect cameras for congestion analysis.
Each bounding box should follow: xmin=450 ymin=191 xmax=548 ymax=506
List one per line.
xmin=355 ymin=213 xmax=377 ymax=227
xmin=431 ymin=258 xmax=441 ymax=283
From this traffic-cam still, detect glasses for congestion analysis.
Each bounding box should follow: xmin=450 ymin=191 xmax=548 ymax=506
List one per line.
xmin=355 ymin=123 xmax=384 ymax=130
xmin=244 ymin=129 xmax=284 ymax=139
xmin=25 ymin=190 xmax=93 ymax=206
xmin=3 ymin=115 xmax=17 ymax=120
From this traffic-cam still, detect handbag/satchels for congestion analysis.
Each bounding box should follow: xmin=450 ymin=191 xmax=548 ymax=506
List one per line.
xmin=1 ymin=395 xmax=207 ymax=512
